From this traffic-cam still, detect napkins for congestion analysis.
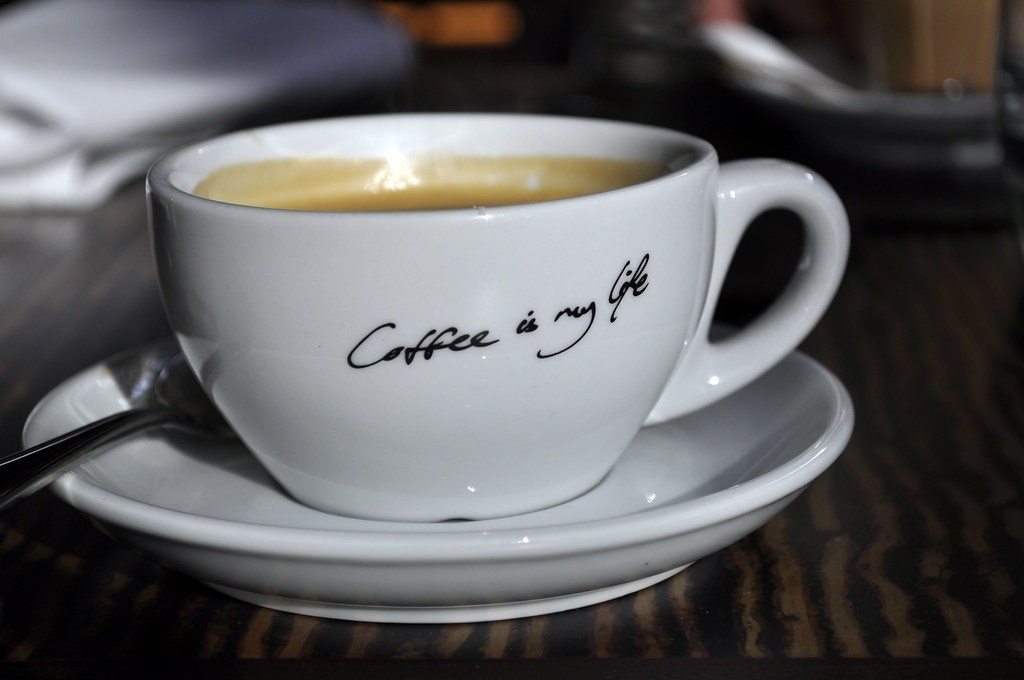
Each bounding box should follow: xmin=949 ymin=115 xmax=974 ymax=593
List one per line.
xmin=1 ymin=0 xmax=406 ymax=209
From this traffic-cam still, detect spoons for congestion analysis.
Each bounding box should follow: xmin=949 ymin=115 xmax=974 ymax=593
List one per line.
xmin=0 ymin=353 xmax=237 ymax=522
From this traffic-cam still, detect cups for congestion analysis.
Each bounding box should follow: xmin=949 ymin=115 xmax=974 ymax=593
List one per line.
xmin=146 ymin=113 xmax=849 ymax=523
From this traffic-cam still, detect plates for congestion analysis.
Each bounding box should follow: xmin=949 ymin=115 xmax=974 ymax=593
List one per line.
xmin=23 ymin=326 xmax=856 ymax=625
